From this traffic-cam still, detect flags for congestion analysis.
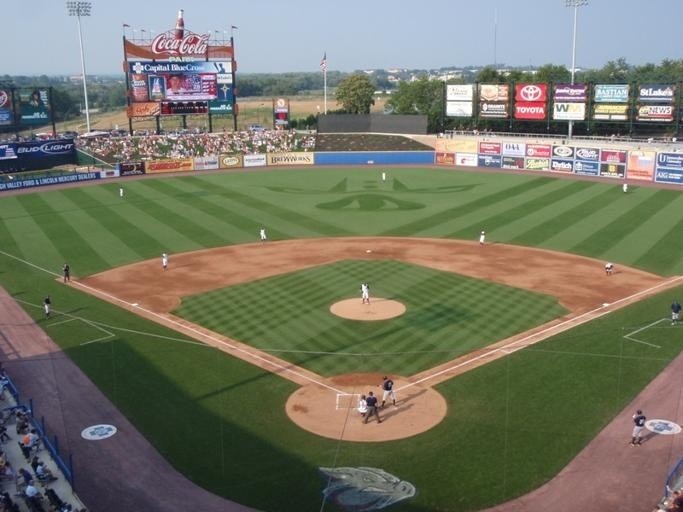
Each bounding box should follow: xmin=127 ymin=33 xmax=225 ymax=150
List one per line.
xmin=318 ymin=55 xmax=327 ymax=71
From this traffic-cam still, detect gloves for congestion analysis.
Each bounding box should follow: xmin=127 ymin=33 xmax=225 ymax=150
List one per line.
xmin=632 ymin=414 xmax=636 ymax=418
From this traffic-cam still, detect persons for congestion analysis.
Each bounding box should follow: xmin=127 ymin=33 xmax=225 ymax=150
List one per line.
xmin=27 ymin=90 xmax=45 ymax=108
xmin=659 ymin=484 xmax=683 ymax=512
xmin=72 ymin=124 xmax=316 ymax=162
xmin=43 ymin=295 xmax=51 ymax=319
xmin=359 ymin=281 xmax=371 ymax=304
xmin=62 ymin=263 xmax=70 ymax=284
xmin=671 ymin=299 xmax=681 ymax=326
xmin=629 ymin=409 xmax=646 ymax=447
xmin=0 ymin=375 xmax=87 ymax=512
xmin=623 ymin=182 xmax=628 ymax=193
xmin=377 ymin=376 xmax=396 ymax=408
xmin=358 ymin=391 xmax=382 ymax=424
xmin=480 ymin=228 xmax=486 ymax=247
xmin=382 ymin=170 xmax=386 ymax=182
xmin=119 ymin=184 xmax=123 ymax=199
xmin=605 ymin=263 xmax=613 ymax=275
xmin=260 ymin=226 xmax=266 ymax=242
xmin=9 ymin=133 xmax=65 ymax=143
xmin=166 ymin=75 xmax=186 ymax=95
xmin=162 ymin=251 xmax=167 ymax=271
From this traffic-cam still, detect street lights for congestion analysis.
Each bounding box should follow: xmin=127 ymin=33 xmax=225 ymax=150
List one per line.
xmin=65 ymin=1 xmax=92 ymax=132
xmin=564 ymin=0 xmax=589 ymax=139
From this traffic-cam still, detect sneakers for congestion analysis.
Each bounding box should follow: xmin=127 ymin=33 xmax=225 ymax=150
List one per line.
xmin=629 ymin=441 xmax=641 ymax=447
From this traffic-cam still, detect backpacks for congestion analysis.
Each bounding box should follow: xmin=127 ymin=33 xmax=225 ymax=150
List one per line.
xmin=23 ymin=436 xmax=30 ymax=444
